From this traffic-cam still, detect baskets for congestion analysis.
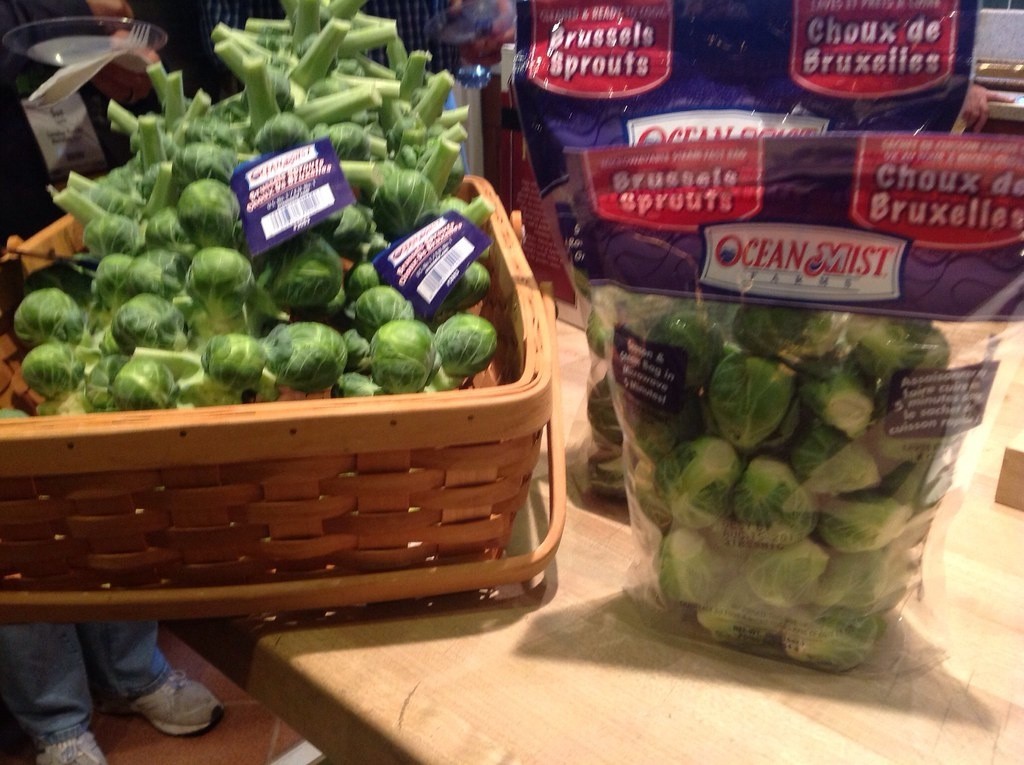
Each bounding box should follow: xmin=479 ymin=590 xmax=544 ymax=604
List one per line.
xmin=0 ymin=170 xmax=567 ymax=625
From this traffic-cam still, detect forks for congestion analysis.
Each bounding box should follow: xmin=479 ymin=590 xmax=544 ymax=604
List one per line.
xmin=28 ymin=24 xmax=150 ymax=102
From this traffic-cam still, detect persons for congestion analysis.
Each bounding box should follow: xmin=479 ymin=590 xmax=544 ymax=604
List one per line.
xmin=0 ymin=0 xmax=225 ymax=765
xmin=199 ymin=0 xmax=517 ymax=173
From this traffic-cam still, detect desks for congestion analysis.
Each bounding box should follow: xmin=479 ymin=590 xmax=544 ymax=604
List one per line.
xmin=988 ymin=87 xmax=1024 ymax=121
xmin=170 ymin=316 xmax=1021 ymax=764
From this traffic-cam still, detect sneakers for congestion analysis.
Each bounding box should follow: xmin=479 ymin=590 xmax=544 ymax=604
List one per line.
xmin=33 ymin=732 xmax=110 ymax=764
xmin=125 ymin=661 xmax=222 ymax=737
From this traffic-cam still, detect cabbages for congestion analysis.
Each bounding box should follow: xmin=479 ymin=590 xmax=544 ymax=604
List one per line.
xmin=0 ymin=27 xmax=498 ymax=423
xmin=571 ymin=268 xmax=962 ymax=673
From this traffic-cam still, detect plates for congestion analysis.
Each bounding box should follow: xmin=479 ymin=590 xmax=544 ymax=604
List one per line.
xmin=3 ymin=16 xmax=168 ymax=68
